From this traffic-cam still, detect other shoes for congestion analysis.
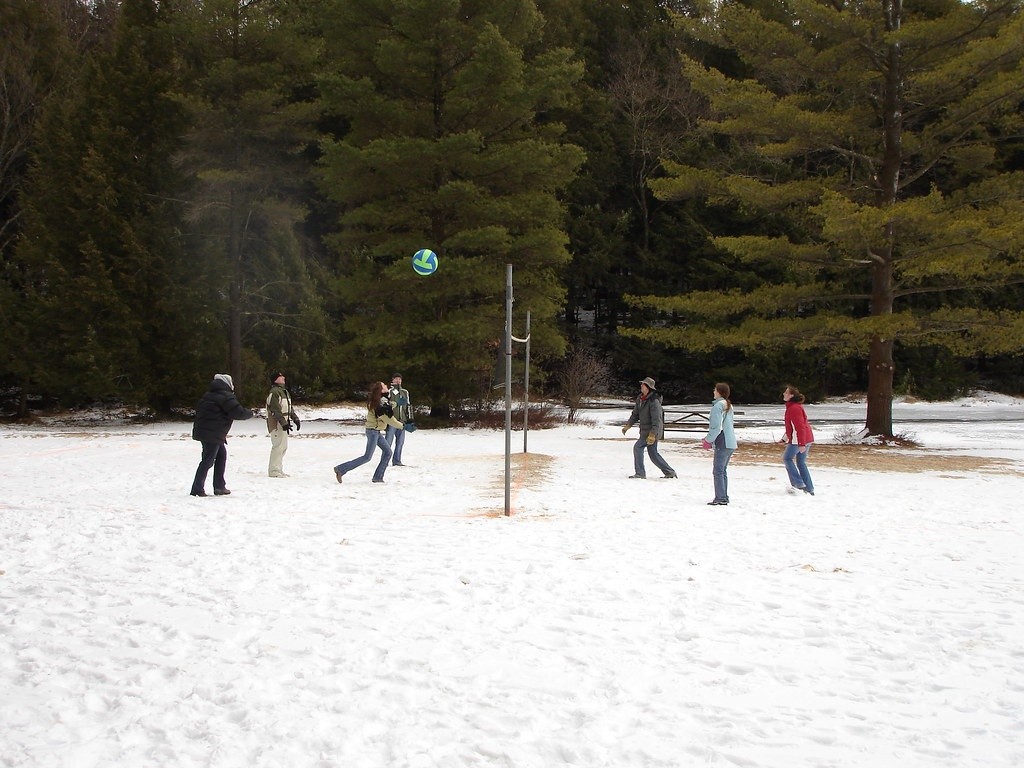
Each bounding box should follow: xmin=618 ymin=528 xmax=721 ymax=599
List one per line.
xmin=629 ymin=474 xmax=646 ymax=479
xmin=707 ymin=501 xmax=727 ymax=505
xmin=373 ymin=480 xmax=385 ymax=483
xmin=809 ymin=492 xmax=814 ymax=496
xmin=273 ymin=471 xmax=287 ymax=477
xmin=789 ymin=487 xmax=807 ymax=494
xmin=190 ymin=489 xmax=207 ymax=497
xmin=214 ymin=487 xmax=231 ymax=495
xmin=393 ymin=462 xmax=405 ymax=466
xmin=334 ymin=467 xmax=342 ymax=483
xmin=660 ymin=475 xmax=678 ymax=478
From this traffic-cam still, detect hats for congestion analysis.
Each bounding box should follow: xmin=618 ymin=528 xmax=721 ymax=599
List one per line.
xmin=393 ymin=373 xmax=402 ymax=378
xmin=270 ymin=373 xmax=282 ymax=382
xmin=639 ymin=377 xmax=656 ymax=390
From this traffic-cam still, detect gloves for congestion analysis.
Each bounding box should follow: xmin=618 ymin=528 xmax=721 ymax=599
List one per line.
xmin=283 ymin=425 xmax=293 ymax=432
xmin=404 ymin=423 xmax=417 ymax=434
xmin=294 ymin=420 xmax=300 ymax=431
xmin=397 ymin=399 xmax=406 ymax=406
xmin=646 ymin=433 xmax=655 ymax=445
xmin=703 ymin=439 xmax=711 ymax=449
xmin=622 ymin=425 xmax=630 ymax=435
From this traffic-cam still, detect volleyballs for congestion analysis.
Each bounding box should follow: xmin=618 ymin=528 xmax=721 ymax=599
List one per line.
xmin=412 ymin=248 xmax=439 ymax=276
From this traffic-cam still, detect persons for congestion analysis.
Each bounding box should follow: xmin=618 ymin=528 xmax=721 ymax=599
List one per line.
xmin=702 ymin=383 xmax=738 ymax=506
xmin=333 ymin=382 xmax=415 ymax=484
xmin=621 ymin=377 xmax=679 ymax=479
xmin=190 ymin=374 xmax=260 ymax=496
xmin=383 ymin=374 xmax=411 ymax=466
xmin=266 ymin=371 xmax=301 ymax=479
xmin=777 ymin=386 xmax=814 ymax=496
